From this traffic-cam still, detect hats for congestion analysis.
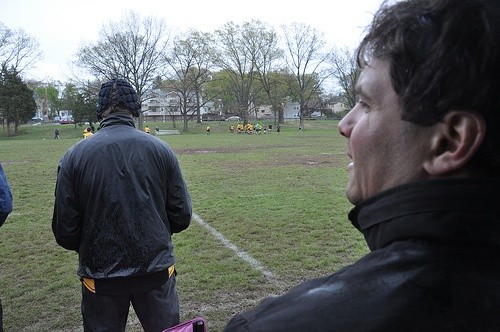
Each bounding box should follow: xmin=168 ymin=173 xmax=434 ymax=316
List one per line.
xmin=96 ymin=78 xmax=141 ymax=119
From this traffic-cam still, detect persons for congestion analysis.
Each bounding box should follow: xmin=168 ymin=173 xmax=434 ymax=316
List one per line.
xmin=0 ymin=163 xmax=13 ymax=332
xmin=54 ymin=122 xmax=303 ymax=139
xmin=223 ymin=0 xmax=500 ymax=332
xmin=51 ymin=78 xmax=192 ymax=332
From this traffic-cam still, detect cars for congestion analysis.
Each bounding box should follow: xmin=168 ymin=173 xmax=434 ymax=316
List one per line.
xmin=224 ymin=116 xmax=241 ymax=122
xmin=31 ymin=114 xmax=74 ymax=125
xmin=311 ymin=112 xmax=326 ymax=119
